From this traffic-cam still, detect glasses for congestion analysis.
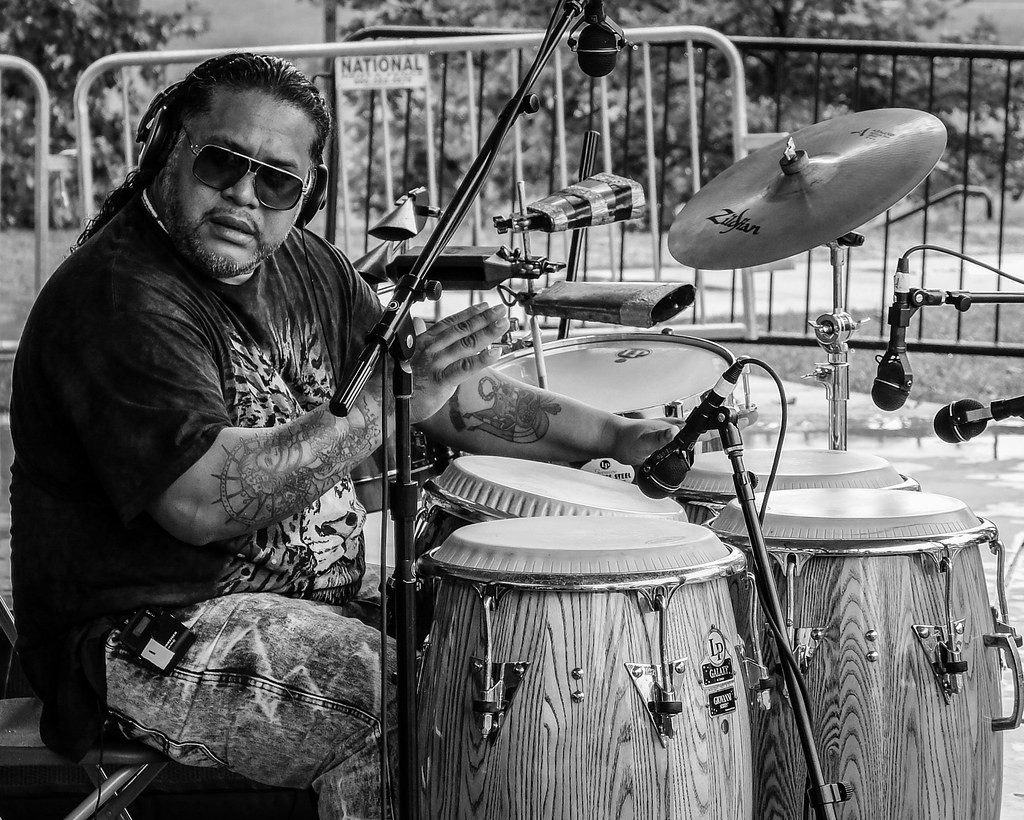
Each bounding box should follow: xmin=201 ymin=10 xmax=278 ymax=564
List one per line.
xmin=180 ymin=120 xmax=313 ymax=212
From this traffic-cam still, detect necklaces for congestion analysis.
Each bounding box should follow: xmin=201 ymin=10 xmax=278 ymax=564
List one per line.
xmin=141 ymin=186 xmax=167 ymax=233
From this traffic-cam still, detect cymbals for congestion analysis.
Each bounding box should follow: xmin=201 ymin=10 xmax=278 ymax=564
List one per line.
xmin=666 ymin=106 xmax=948 ymax=272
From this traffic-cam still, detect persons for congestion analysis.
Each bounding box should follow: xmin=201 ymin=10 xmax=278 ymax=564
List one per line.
xmin=8 ymin=53 xmax=761 ymax=820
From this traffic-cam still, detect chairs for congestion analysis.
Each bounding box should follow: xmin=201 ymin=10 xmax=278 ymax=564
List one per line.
xmin=0 ymin=595 xmax=168 ymax=820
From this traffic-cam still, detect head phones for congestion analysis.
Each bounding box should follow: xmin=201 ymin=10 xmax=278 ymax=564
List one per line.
xmin=136 ymin=80 xmax=329 ymax=229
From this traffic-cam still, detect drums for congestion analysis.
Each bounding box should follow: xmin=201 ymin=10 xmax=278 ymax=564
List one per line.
xmin=708 ymin=488 xmax=1024 ymax=820
xmin=413 ymin=514 xmax=757 ymax=820
xmin=484 ymin=329 xmax=739 ymax=457
xmin=666 ymin=448 xmax=921 ymax=530
xmin=387 ymin=454 xmax=691 ymax=652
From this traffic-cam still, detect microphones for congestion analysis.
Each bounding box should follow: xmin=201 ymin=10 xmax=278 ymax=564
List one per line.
xmin=871 ymin=257 xmax=913 ymax=411
xmin=386 ymin=245 xmax=566 ymax=292
xmin=934 ymin=396 xmax=1024 ymax=444
xmin=577 ymin=0 xmax=620 ymax=78
xmin=637 ymin=362 xmax=744 ymax=500
